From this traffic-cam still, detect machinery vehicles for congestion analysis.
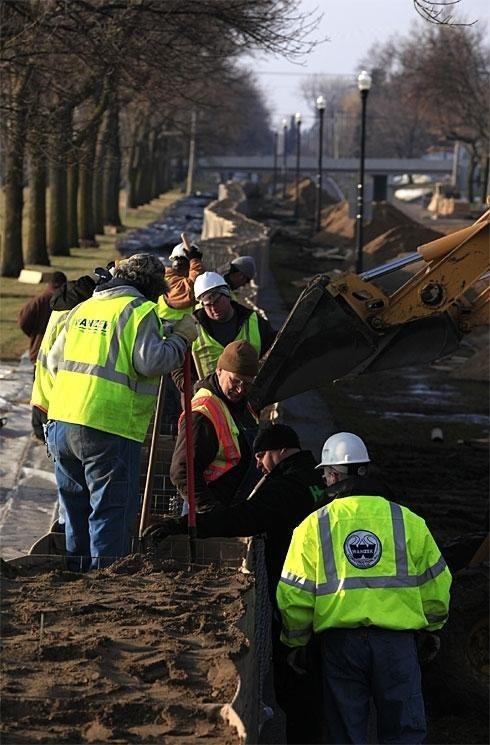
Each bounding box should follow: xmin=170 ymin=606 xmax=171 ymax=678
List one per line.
xmin=242 ymin=202 xmax=489 ymax=413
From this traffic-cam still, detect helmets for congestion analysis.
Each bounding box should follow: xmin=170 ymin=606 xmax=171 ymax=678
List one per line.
xmin=169 ymin=241 xmax=200 ymax=262
xmin=314 ymin=431 xmax=371 ymax=470
xmin=194 ymin=271 xmax=228 ymax=301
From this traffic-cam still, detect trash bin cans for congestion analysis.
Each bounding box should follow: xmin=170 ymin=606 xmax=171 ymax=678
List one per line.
xmin=421 ymin=196 xmax=432 ymax=210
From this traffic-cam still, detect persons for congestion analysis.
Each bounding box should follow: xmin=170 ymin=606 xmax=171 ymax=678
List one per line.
xmin=143 ymin=421 xmax=328 ymax=745
xmin=15 ymin=272 xmax=69 ymax=360
xmin=26 ymin=259 xmax=121 ymax=535
xmin=168 ymin=340 xmax=262 ymax=538
xmin=169 ymin=271 xmax=276 ymax=406
xmin=156 ymin=237 xmax=206 ymax=436
xmin=193 ymin=253 xmax=258 ymax=310
xmin=276 ymin=430 xmax=454 ymax=744
xmin=26 ymin=252 xmax=198 ymax=569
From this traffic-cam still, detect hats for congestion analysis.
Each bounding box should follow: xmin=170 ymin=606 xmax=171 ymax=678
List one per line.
xmin=252 ymin=424 xmax=301 ymax=459
xmin=232 ymin=256 xmax=255 ymax=279
xmin=217 ymin=340 xmax=258 ymax=377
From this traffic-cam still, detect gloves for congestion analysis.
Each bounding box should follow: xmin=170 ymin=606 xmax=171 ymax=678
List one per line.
xmin=183 ymin=246 xmax=201 ymax=259
xmin=173 ymin=314 xmax=198 ymax=343
xmin=416 ymin=631 xmax=440 ymax=665
xmin=281 ymin=643 xmax=307 ymax=675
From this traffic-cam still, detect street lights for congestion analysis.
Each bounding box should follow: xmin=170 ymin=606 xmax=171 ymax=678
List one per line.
xmin=270 ymin=95 xmax=332 ymax=236
xmin=351 ymin=69 xmax=374 ymax=276
